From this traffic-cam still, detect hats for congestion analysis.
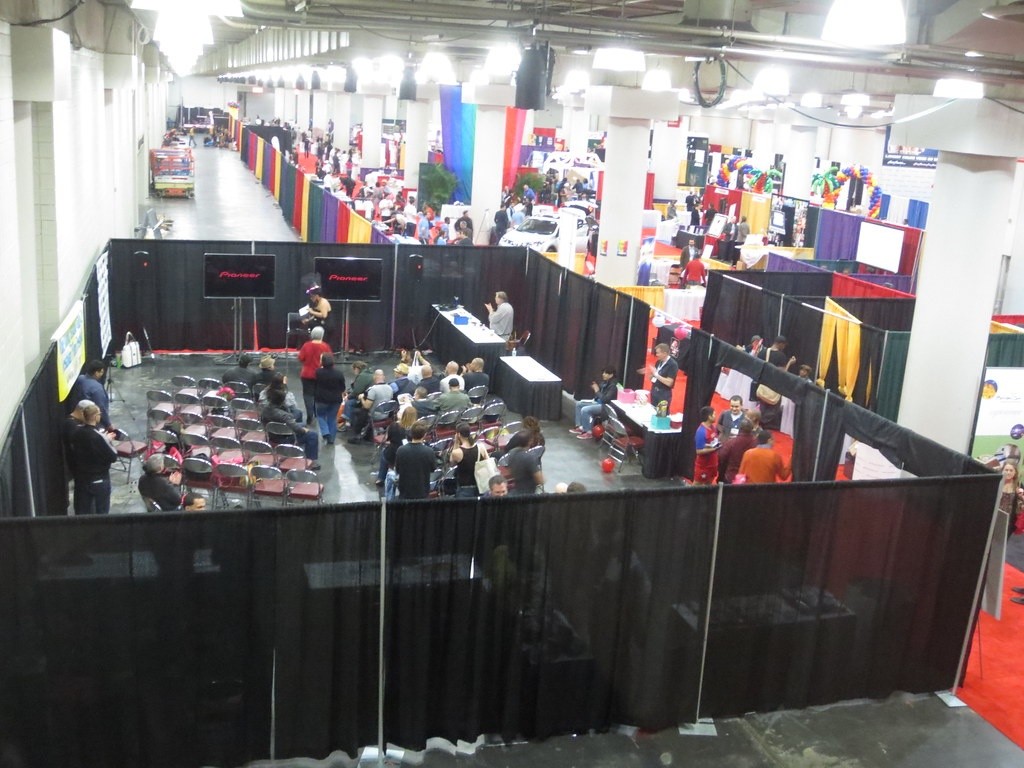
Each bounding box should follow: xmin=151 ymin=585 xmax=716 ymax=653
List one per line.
xmin=393 ymin=363 xmax=409 ymax=375
xmin=271 ymin=390 xmax=285 ymax=404
xmin=448 ymin=378 xmax=459 ymax=385
xmin=258 ymin=355 xmax=275 ymax=368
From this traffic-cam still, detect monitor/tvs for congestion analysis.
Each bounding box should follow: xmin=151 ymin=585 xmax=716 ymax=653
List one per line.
xmin=314 ymin=257 xmax=383 ymax=302
xmin=203 ymin=253 xmax=276 ymax=299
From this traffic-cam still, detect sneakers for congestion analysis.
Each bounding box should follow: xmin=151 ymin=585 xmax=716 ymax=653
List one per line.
xmin=568 ymin=426 xmax=583 ymax=435
xmin=577 ymin=432 xmax=593 ymax=439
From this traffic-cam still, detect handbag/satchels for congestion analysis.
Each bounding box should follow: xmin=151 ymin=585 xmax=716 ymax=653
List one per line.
xmin=121 ymin=332 xmax=142 ymax=368
xmin=755 ymin=383 xmax=781 ymax=406
xmin=474 ymin=442 xmax=502 ymax=495
xmin=407 ymin=350 xmax=424 ymax=385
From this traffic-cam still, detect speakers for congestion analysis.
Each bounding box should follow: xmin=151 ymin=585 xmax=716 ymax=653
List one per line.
xmin=134 ymin=251 xmax=150 ymax=274
xmin=408 ymin=254 xmax=424 ymax=280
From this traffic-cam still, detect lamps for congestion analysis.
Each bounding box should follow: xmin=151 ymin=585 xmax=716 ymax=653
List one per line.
xmin=130 ymin=0 xmax=986 ymax=122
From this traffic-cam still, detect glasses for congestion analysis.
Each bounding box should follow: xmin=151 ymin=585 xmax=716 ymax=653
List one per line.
xmin=96 ymin=411 xmax=103 ymax=417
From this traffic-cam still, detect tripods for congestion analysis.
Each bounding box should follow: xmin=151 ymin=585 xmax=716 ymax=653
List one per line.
xmin=105 ymin=362 xmax=135 ymax=421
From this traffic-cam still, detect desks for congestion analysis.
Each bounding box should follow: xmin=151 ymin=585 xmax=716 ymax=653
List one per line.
xmin=610 ymin=399 xmax=683 ymax=478
xmin=430 ymin=303 xmax=481 ymax=363
xmin=444 ymin=322 xmax=506 ymax=375
xmin=675 ymin=230 xmax=768 ymax=272
xmin=493 ymin=356 xmax=563 ymax=421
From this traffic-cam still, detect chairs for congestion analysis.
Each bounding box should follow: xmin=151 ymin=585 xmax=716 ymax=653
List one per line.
xmin=110 ymin=375 xmax=324 ymax=511
xmin=370 ymin=383 xmax=545 ymax=499
xmin=506 ymin=330 xmax=532 ymax=356
xmin=600 ymin=404 xmax=632 ymax=451
xmin=606 ymin=415 xmax=645 ymax=472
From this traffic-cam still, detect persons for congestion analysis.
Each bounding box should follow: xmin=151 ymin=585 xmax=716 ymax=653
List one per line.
xmin=665 ymin=191 xmax=718 ymax=226
xmin=65 ymin=359 xmax=118 ymax=515
xmin=569 ymin=365 xmax=618 ymax=439
xmin=273 ymin=116 xmax=474 ymax=246
xmin=723 ymin=215 xmax=749 ymax=262
xmin=746 ymin=334 xmax=813 ymax=383
xmin=223 ymin=354 xmax=322 ymax=470
xmin=485 ymin=290 xmax=514 ymax=341
xmin=998 ymin=460 xmax=1024 ymax=542
xmin=188 ymin=126 xmax=196 ymax=145
xmin=298 ymin=281 xmax=346 ymax=444
xmin=642 ymin=343 xmax=678 ymax=415
xmin=679 ymin=237 xmax=707 ymax=285
xmin=493 ymin=176 xmax=589 ymax=246
xmin=138 ymin=453 xmax=207 ymax=511
xmin=210 ymin=124 xmax=229 ymax=142
xmin=692 ymin=395 xmax=791 ymax=485
xmin=337 ymin=350 xmax=545 ymax=500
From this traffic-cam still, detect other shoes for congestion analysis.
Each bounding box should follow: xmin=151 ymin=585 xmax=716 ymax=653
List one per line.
xmin=337 ymin=423 xmax=348 ymax=432
xmin=375 ymin=480 xmax=384 ymax=486
xmin=306 ymin=418 xmax=314 ymax=426
xmin=347 ymin=435 xmax=361 ymax=443
xmin=323 ymin=434 xmax=334 ymax=447
xmin=306 ymin=462 xmax=321 ymax=471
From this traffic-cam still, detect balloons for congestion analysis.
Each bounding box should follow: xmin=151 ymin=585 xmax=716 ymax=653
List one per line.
xmin=716 ymin=155 xmax=782 ymax=195
xmin=811 ymin=162 xmax=882 ymax=219
